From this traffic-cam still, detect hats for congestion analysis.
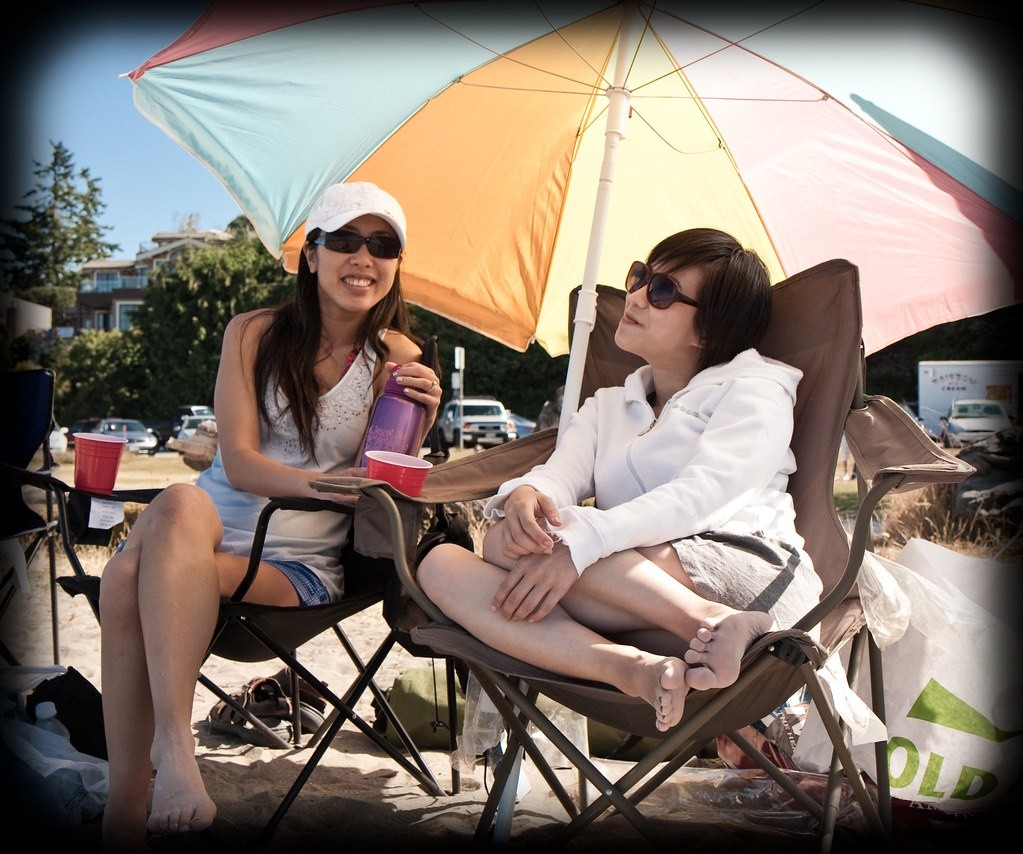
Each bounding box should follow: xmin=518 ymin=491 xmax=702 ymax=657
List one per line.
xmin=305 ymin=182 xmax=408 ymax=253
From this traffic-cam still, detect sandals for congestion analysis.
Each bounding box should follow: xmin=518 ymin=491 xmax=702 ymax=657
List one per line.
xmin=208 ymin=676 xmax=294 ymax=746
xmin=241 ymin=665 xmax=325 ymax=732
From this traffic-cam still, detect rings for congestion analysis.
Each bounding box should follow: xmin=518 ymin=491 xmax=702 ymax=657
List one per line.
xmin=428 ymin=381 xmax=437 ymax=394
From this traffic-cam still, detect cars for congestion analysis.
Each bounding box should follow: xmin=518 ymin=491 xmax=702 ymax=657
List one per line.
xmin=939 ymin=397 xmax=1016 ymax=450
xmin=60 ymin=402 xmax=220 ymax=458
xmin=509 ymin=412 xmax=540 ymax=436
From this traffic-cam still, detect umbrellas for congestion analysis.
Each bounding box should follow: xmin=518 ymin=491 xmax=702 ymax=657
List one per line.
xmin=123 ymin=5 xmax=1005 ymax=828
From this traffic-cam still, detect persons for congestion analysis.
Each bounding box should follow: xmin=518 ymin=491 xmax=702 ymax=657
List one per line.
xmin=12 ymin=331 xmax=59 ymax=560
xmin=100 ymin=182 xmax=443 ymax=829
xmin=939 ymin=415 xmax=960 ymax=449
xmin=411 ymin=223 xmax=826 ymax=737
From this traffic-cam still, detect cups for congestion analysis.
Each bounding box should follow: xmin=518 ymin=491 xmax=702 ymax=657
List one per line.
xmin=72 ymin=429 xmax=129 ymax=493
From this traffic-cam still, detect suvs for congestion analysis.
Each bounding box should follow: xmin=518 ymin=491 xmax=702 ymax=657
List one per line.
xmin=439 ymin=396 xmax=520 ymax=449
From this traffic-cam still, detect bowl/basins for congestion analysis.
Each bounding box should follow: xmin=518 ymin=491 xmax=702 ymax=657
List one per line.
xmin=362 ymin=450 xmax=432 ymax=497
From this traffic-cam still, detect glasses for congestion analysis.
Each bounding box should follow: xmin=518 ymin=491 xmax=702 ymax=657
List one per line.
xmin=624 ymin=260 xmax=699 ymax=311
xmin=313 ymin=229 xmax=401 ymax=258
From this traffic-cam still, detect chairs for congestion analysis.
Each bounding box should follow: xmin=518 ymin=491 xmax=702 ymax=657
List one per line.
xmin=407 ymin=254 xmax=979 ymax=853
xmin=49 ymin=331 xmax=446 ymax=824
xmin=1 ymin=369 xmax=102 ymax=670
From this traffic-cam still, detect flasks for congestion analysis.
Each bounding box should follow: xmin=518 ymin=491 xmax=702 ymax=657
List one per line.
xmin=360 ymin=361 xmax=422 ymax=469
